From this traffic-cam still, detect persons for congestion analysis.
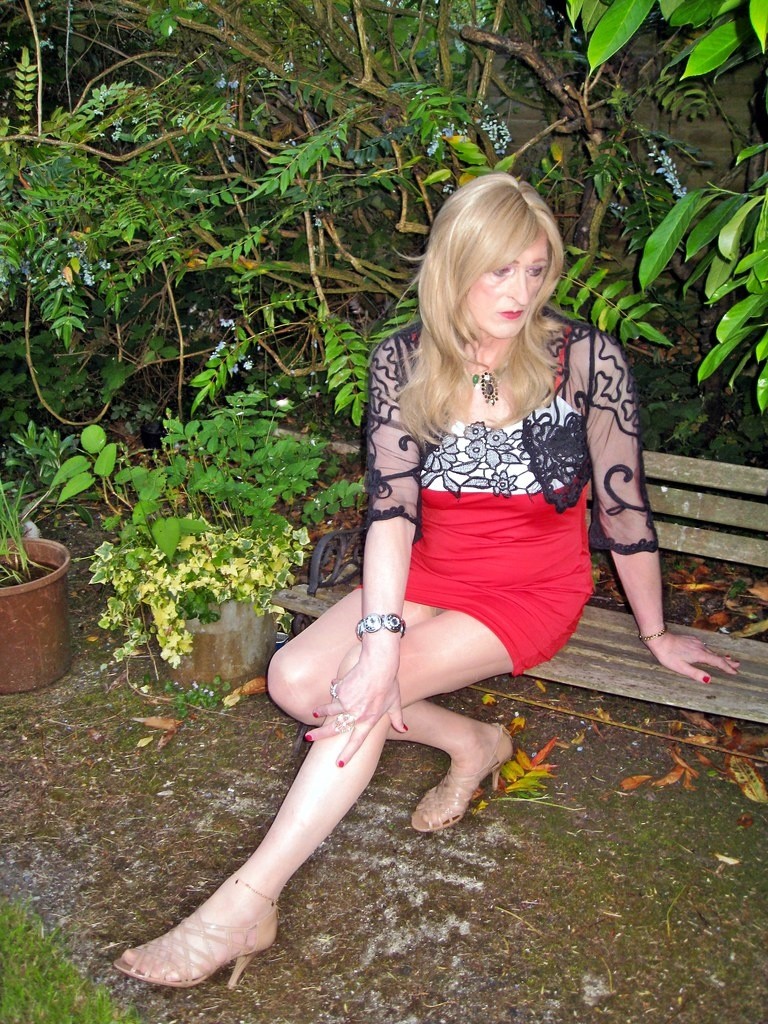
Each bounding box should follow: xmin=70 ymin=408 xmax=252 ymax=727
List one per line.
xmin=113 ymin=172 xmax=740 ymax=991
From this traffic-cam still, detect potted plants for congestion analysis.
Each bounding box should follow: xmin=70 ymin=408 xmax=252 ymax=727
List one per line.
xmin=0 ymin=470 xmax=71 ymax=694
xmin=60 ymin=388 xmax=368 ymax=697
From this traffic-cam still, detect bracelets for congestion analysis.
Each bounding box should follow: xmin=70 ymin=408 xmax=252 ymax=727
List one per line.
xmin=638 ymin=624 xmax=667 ymax=640
xmin=357 ymin=613 xmax=406 ymax=641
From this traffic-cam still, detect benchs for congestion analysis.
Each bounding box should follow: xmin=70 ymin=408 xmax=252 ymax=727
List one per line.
xmin=269 ymin=449 xmax=768 ymax=764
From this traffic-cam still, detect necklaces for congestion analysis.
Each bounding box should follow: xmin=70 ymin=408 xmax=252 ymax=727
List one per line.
xmin=470 ymin=365 xmax=508 ymax=403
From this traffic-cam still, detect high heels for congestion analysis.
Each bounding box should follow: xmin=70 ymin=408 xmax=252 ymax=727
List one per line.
xmin=114 ymin=874 xmax=279 ymax=990
xmin=412 ymin=724 xmax=512 ymax=832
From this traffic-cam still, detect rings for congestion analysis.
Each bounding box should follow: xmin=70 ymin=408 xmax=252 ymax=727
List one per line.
xmin=330 ymin=681 xmax=342 ymax=698
xmin=335 ymin=714 xmax=355 ymax=733
xmin=704 ymin=643 xmax=707 ymax=648
